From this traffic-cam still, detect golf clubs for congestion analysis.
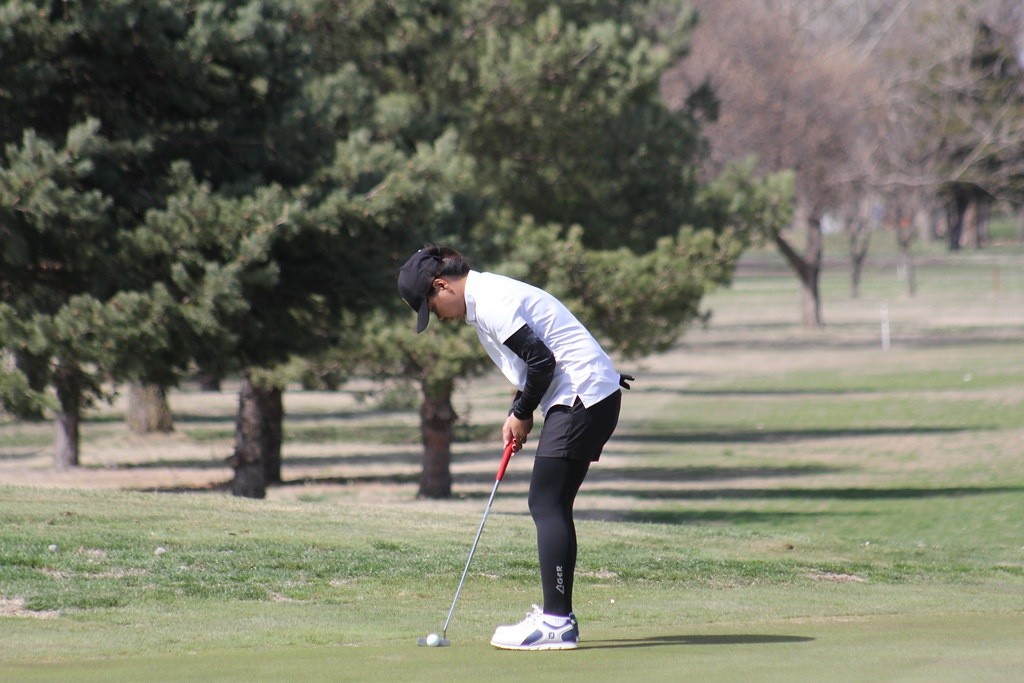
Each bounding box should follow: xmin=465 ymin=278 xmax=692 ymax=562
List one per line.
xmin=418 ymin=435 xmax=518 ymax=647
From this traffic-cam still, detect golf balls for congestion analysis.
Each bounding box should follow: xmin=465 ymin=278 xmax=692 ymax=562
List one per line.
xmin=427 ymin=634 xmax=440 ymax=647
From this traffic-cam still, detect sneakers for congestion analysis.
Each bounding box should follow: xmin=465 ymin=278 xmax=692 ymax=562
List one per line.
xmin=490 ymin=604 xmax=581 ymax=649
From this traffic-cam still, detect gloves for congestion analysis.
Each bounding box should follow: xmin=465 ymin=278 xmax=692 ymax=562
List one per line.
xmin=620 ymin=374 xmax=635 ymax=390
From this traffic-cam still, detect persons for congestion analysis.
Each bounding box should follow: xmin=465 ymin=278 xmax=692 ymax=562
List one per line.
xmin=398 ymin=243 xmax=635 ymax=650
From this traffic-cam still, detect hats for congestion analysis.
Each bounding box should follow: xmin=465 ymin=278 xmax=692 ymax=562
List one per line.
xmin=397 ymin=248 xmax=442 ymax=333
